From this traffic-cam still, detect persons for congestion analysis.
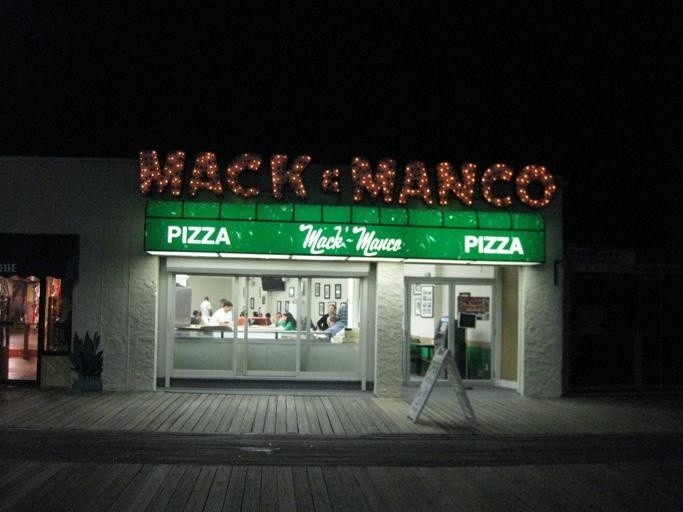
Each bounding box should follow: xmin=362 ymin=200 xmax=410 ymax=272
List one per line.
xmin=279 ymin=312 xmax=297 ymax=330
xmin=259 ymin=313 xmax=263 ymax=318
xmin=265 ymin=312 xmax=272 ymax=325
xmin=272 ymin=312 xmax=282 ymax=327
xmin=253 ymin=312 xmax=261 ymax=325
xmin=326 ymin=316 xmax=345 ymax=337
xmin=190 ymin=310 xmax=205 ymax=324
xmin=220 ymin=299 xmax=227 ymax=308
xmin=200 ymin=296 xmax=212 ymax=324
xmin=210 ymin=301 xmax=233 ymax=323
xmin=238 ymin=311 xmax=248 ymax=325
xmin=317 ymin=305 xmax=340 ymax=331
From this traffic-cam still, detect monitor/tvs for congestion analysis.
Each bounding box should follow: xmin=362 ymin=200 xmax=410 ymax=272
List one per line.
xmin=262 ymin=277 xmax=285 ymax=291
xmin=439 ymin=322 xmax=447 ymax=333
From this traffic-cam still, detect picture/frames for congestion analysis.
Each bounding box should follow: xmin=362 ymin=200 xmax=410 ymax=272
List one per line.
xmin=414 ymin=284 xmax=434 ymax=317
xmin=235 ymin=278 xmax=342 ymax=317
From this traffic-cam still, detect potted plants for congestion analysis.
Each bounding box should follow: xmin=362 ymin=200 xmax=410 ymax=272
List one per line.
xmin=69 ymin=330 xmax=103 ymax=389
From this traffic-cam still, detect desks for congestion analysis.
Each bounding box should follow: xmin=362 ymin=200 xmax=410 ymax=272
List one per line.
xmin=410 ymin=342 xmax=435 ymax=366
